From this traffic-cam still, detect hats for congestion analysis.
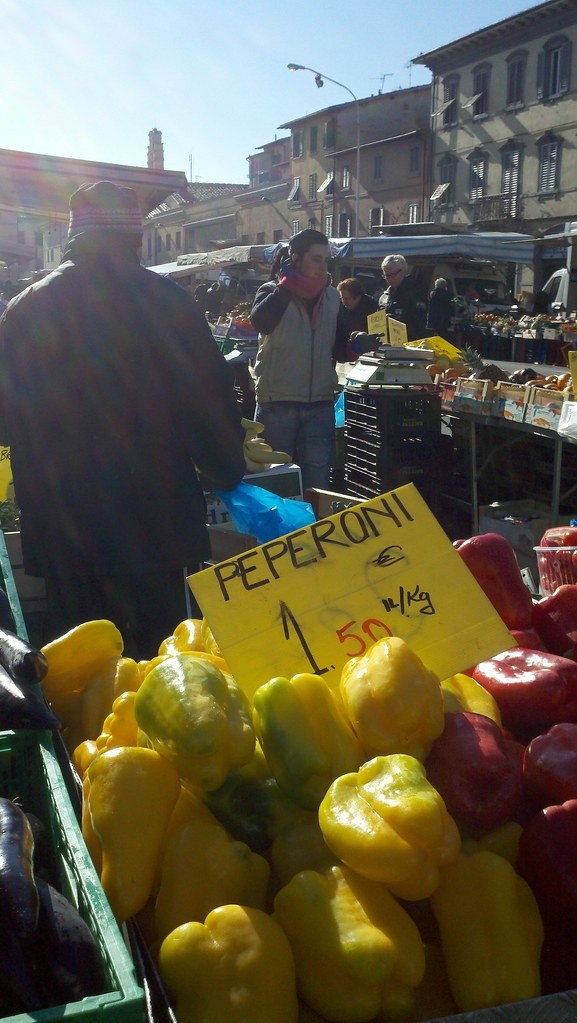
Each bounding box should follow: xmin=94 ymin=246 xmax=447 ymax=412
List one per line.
xmin=67 ymin=180 xmax=143 ymax=246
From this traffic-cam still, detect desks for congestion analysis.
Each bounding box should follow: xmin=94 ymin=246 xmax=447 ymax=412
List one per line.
xmin=440 ymin=408 xmax=577 ymax=537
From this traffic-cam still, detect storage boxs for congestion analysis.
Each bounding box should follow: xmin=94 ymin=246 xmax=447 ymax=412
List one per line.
xmin=515 ymin=328 xmax=577 ymax=342
xmin=0 ymin=385 xmax=440 ymax=1023
xmin=213 ymin=316 xmax=262 ymax=354
xmin=452 ymin=377 xmax=577 ymax=440
xmin=477 ymin=502 xmax=572 ymax=585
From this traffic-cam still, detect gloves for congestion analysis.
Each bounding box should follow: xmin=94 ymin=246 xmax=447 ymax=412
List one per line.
xmin=354 ymin=332 xmax=385 ymax=353
xmin=280 ymin=254 xmax=298 ymax=276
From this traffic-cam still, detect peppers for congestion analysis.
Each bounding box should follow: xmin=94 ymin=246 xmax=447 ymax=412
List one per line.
xmin=36 ymin=523 xmax=577 ymax=1023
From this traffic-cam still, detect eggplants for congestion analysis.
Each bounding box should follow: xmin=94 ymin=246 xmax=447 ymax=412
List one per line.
xmin=0 ymin=628 xmax=100 ymax=1014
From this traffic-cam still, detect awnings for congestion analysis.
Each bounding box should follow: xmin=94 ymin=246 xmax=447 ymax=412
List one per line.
xmin=317 ymin=178 xmax=333 ymax=192
xmin=461 ymin=89 xmax=486 ymax=108
xmin=431 ymin=98 xmax=456 ymax=116
xmin=431 ymin=182 xmax=451 ymax=200
xmin=287 ymin=186 xmax=298 ymax=200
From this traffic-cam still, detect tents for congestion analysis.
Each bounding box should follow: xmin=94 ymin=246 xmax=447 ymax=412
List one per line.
xmin=145 ymin=226 xmax=570 ymax=277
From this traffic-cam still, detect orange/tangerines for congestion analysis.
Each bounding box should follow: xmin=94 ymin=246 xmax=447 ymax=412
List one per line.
xmin=426 ymin=363 xmax=460 ymax=379
xmin=525 ymin=373 xmax=573 ymax=393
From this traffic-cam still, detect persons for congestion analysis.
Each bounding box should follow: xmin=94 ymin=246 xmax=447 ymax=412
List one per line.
xmin=0 ymin=180 xmax=245 ymax=659
xmin=337 ymin=277 xmax=378 ymax=333
xmin=526 ymin=292 xmax=548 ymax=363
xmin=426 ymin=278 xmax=456 ymax=339
xmin=5 ymin=281 xmax=13 ymax=292
xmin=193 ymin=278 xmax=241 ymax=315
xmin=249 ymin=228 xmax=384 ymax=491
xmin=379 ymin=254 xmax=429 ymax=341
xmin=465 ymin=282 xmax=480 ymax=298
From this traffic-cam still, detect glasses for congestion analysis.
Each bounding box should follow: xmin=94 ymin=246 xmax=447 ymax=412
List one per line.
xmin=381 ymin=269 xmax=401 ymax=279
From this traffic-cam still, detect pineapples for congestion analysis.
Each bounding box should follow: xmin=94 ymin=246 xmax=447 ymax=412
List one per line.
xmin=456 ymin=342 xmax=509 ymax=385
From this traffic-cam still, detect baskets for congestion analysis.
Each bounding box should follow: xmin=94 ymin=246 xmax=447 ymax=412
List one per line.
xmin=533 ymin=545 xmax=577 ymax=595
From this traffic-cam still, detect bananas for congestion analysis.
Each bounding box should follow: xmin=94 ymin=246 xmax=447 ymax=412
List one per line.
xmin=241 ymin=417 xmax=293 ymax=473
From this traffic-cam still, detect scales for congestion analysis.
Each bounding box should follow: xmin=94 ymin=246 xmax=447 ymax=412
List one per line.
xmin=224 ymin=338 xmax=258 ymax=364
xmin=345 ymin=347 xmax=435 ymax=388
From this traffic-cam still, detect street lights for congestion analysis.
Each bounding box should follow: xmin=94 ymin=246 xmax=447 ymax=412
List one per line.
xmin=288 ymin=63 xmax=359 ymax=239
